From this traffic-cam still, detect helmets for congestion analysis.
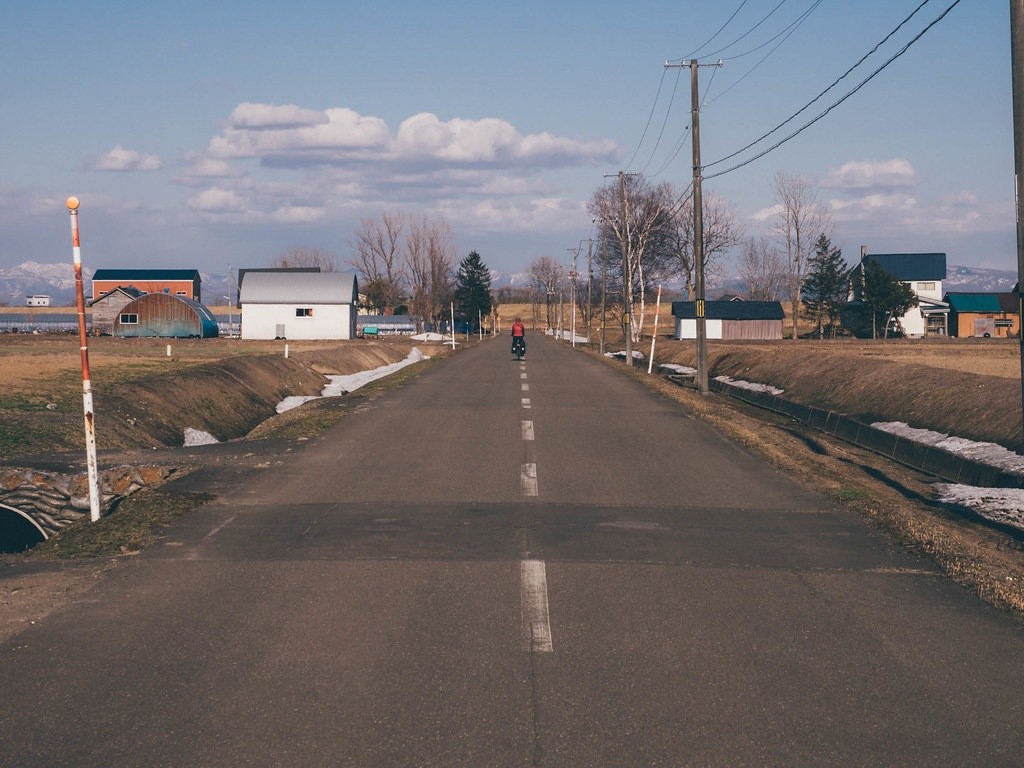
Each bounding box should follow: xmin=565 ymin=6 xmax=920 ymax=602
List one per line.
xmin=515 ymin=318 xmax=521 ymax=322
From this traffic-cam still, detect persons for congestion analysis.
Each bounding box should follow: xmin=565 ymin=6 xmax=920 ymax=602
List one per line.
xmin=511 ymin=318 xmax=525 ymax=343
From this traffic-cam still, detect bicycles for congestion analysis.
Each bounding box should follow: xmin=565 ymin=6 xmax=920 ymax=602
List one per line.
xmin=512 ymin=334 xmax=526 ymax=361
